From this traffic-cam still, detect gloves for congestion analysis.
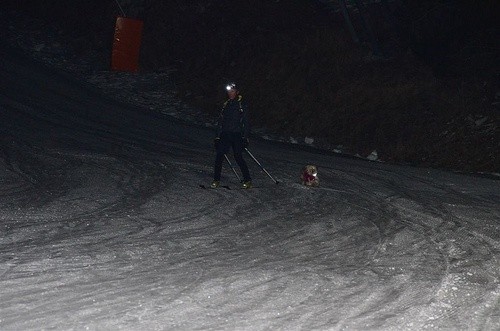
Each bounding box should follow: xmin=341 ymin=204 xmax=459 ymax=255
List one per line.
xmin=240 ymin=136 xmax=249 ymax=151
xmin=214 ymin=138 xmax=222 ymax=149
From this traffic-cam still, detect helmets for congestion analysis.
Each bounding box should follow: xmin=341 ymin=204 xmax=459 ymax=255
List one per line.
xmin=224 ymin=82 xmax=236 ymax=89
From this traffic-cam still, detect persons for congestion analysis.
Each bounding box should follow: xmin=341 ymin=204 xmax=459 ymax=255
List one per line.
xmin=209 ymin=79 xmax=256 ymax=189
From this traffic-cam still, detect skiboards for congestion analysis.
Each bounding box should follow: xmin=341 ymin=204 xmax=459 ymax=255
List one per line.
xmin=200 ymin=183 xmax=272 ymax=190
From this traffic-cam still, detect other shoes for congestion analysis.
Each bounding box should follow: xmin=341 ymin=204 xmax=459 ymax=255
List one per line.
xmin=240 ymin=180 xmax=253 ymax=189
xmin=210 ymin=180 xmax=220 ymax=189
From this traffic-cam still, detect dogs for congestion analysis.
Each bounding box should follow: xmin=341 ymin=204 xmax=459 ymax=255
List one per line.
xmin=300 ymin=164 xmax=320 ymax=187
